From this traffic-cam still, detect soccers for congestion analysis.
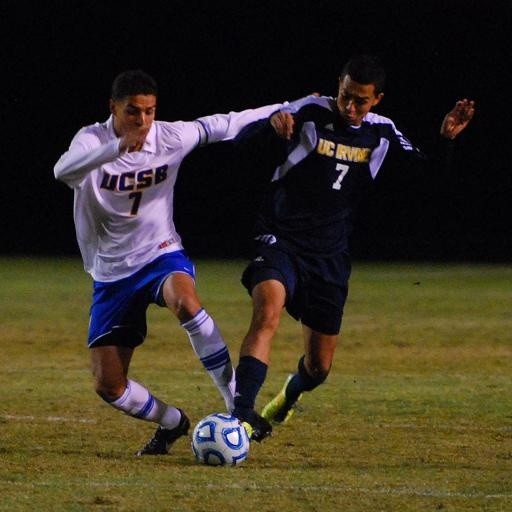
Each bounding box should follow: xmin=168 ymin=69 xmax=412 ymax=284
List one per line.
xmin=190 ymin=412 xmax=249 ymax=468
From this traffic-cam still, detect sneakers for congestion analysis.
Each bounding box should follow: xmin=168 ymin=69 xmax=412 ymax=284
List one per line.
xmin=229 ymin=374 xmax=303 ymax=444
xmin=137 ymin=408 xmax=191 ymax=456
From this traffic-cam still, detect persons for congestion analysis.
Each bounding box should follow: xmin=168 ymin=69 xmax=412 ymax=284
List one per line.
xmin=233 ymin=51 xmax=474 ymax=439
xmin=51 ymin=65 xmax=321 ymax=458
